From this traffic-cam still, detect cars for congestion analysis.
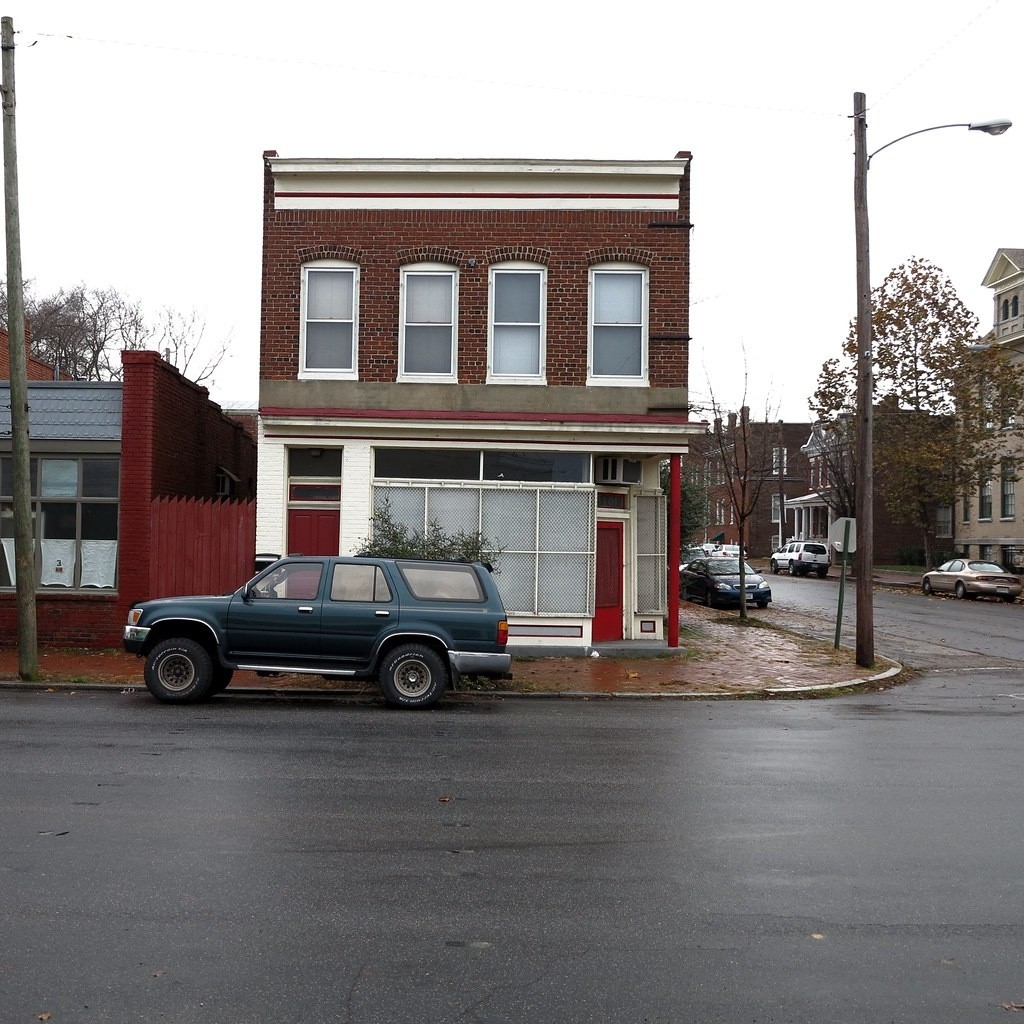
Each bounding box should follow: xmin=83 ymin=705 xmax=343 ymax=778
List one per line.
xmin=921 ymin=558 xmax=1024 ymax=603
xmin=679 ymin=556 xmax=772 ymax=609
xmin=680 ymin=543 xmax=748 ymax=564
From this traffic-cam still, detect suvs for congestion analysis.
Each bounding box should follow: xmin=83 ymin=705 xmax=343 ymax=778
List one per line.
xmin=121 ymin=551 xmax=515 ymax=709
xmin=770 ymin=542 xmax=831 ymax=580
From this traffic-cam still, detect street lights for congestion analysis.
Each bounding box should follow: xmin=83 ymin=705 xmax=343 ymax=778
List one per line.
xmin=852 ymin=91 xmax=1012 ymax=670
